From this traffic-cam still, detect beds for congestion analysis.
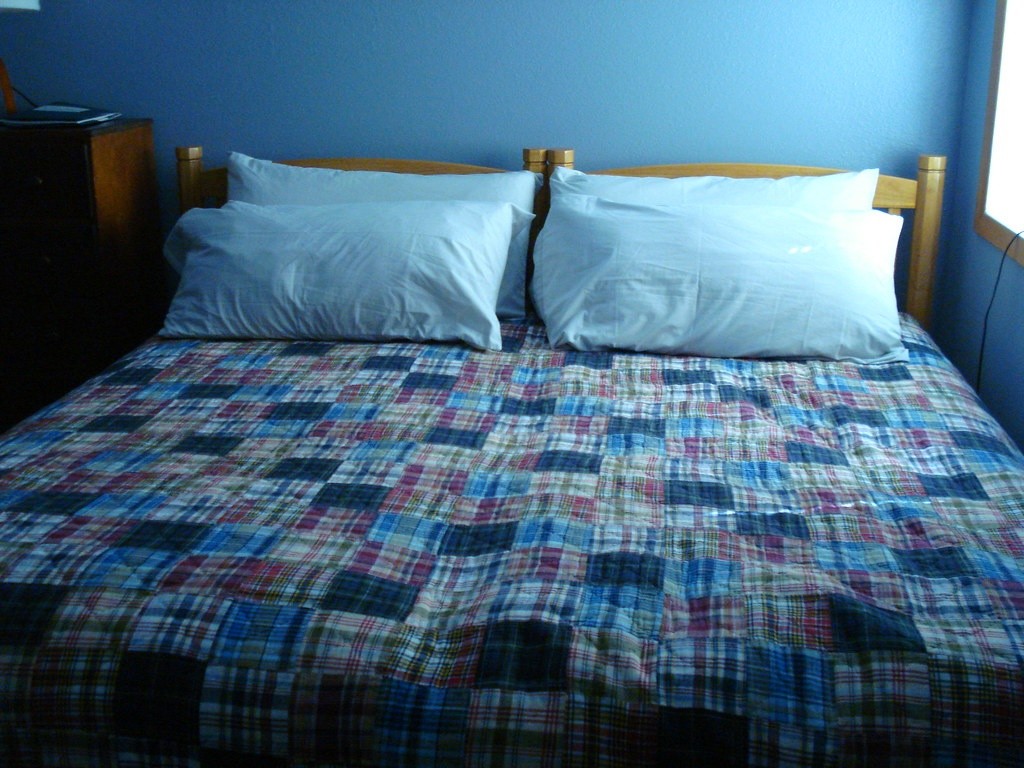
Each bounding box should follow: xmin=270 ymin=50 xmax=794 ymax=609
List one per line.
xmin=0 ymin=145 xmax=1024 ymax=768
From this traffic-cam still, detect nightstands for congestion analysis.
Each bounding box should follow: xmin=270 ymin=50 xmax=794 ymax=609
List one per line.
xmin=0 ymin=119 xmax=161 ymax=412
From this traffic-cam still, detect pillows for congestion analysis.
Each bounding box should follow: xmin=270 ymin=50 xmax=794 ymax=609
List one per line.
xmin=225 ymin=151 xmax=544 ymax=326
xmin=529 ymin=194 xmax=911 ymax=366
xmin=550 ymin=166 xmax=881 ymax=209
xmin=157 ymin=200 xmax=535 ymax=352
